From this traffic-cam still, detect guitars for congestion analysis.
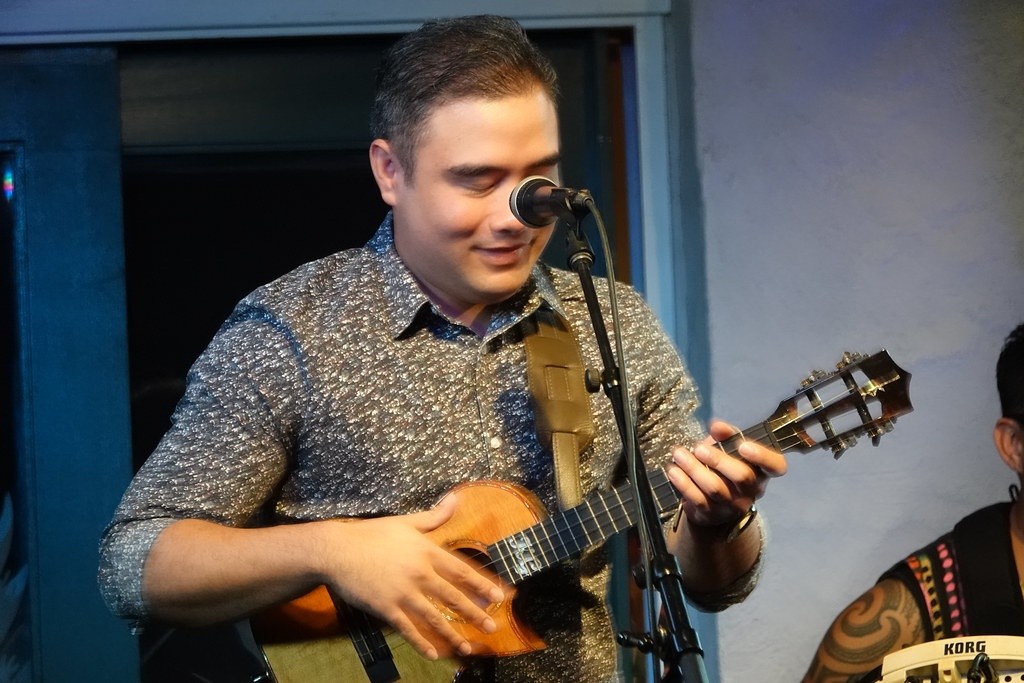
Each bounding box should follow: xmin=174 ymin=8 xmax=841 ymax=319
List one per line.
xmin=250 ymin=348 xmax=917 ymax=683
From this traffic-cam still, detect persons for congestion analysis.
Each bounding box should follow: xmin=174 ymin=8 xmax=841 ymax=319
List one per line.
xmin=801 ymin=320 xmax=1024 ymax=683
xmin=94 ymin=11 xmax=760 ymax=682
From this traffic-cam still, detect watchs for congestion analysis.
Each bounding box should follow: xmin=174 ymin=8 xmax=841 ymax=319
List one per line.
xmin=684 ymin=507 xmax=758 ymax=546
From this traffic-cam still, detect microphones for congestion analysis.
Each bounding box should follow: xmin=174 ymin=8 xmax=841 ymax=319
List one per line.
xmin=251 ymin=671 xmax=271 ymax=683
xmin=508 ymin=174 xmax=593 ymax=229
